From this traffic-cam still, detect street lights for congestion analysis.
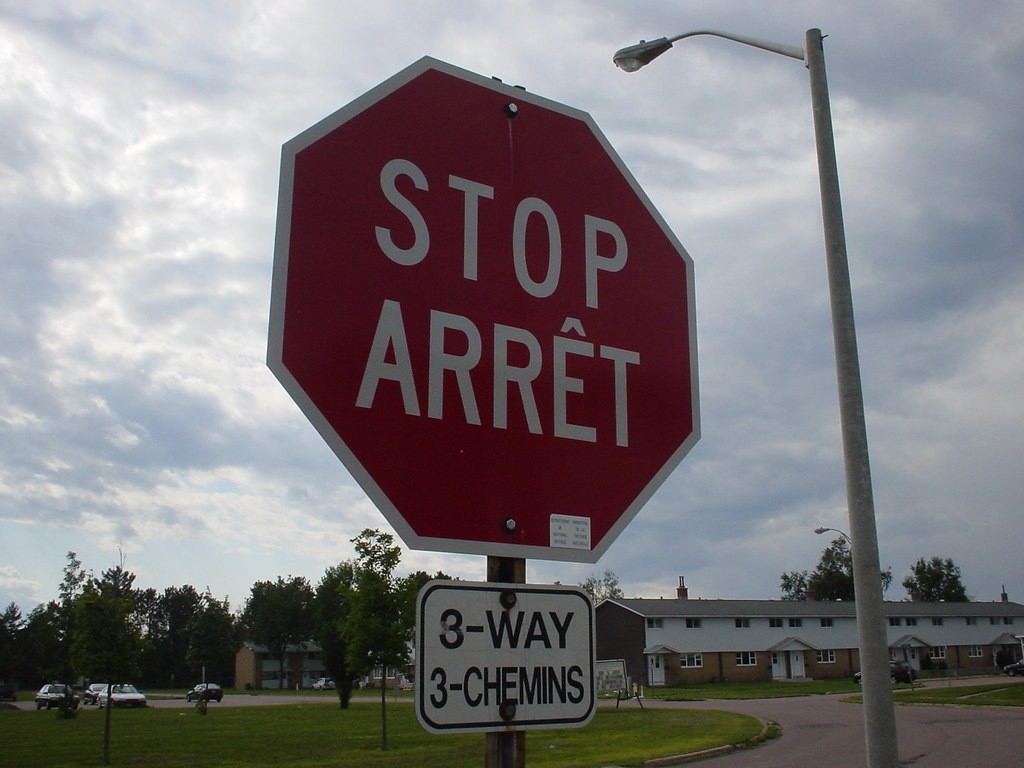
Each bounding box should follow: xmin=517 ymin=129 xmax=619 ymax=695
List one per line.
xmin=612 ymin=28 xmax=900 ymax=768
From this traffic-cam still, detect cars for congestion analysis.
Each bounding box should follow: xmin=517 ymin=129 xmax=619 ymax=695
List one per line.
xmin=96 ymin=684 xmax=146 ymax=710
xmin=35 ymin=683 xmax=80 ymax=710
xmin=1003 ymin=659 xmax=1024 ymax=676
xmin=187 ymin=683 xmax=223 ymax=702
xmin=84 ymin=684 xmax=108 ymax=705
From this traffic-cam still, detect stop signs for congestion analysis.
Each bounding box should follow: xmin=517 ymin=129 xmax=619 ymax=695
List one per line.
xmin=261 ymin=52 xmax=702 ymax=566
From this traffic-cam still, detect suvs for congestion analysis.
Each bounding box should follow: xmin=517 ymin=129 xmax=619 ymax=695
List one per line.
xmin=312 ymin=678 xmax=335 ymax=691
xmin=853 ymin=660 xmax=916 ymax=684
